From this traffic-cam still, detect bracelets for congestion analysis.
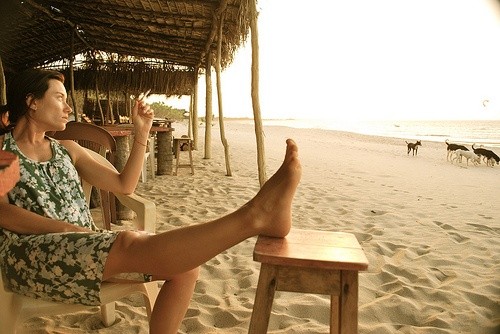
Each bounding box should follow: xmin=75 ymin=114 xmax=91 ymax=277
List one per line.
xmin=134 ymin=136 xmax=148 ymax=147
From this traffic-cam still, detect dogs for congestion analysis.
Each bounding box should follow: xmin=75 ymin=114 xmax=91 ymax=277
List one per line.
xmin=445 ymin=139 xmax=500 ymax=167
xmin=405 ymin=140 xmax=422 ymax=157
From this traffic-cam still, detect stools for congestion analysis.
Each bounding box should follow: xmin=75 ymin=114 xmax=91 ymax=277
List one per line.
xmin=248 ymin=226 xmax=369 ymax=334
xmin=174 ymin=138 xmax=194 ymax=176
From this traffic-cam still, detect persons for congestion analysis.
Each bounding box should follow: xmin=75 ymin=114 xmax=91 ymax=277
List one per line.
xmin=0 ymin=69 xmax=302 ymax=334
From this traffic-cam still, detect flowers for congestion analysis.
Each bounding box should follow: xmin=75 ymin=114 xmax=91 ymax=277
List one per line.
xmin=129 ymin=95 xmax=135 ymax=100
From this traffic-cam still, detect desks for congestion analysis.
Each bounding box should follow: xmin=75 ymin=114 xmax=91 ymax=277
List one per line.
xmin=100 ymin=126 xmax=131 ymax=137
xmin=121 ymin=127 xmax=174 ymax=131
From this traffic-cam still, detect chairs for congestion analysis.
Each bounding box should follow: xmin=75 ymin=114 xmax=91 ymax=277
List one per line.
xmin=0 ymin=121 xmax=157 ymax=334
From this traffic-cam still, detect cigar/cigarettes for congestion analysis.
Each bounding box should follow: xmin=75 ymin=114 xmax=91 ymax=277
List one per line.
xmin=137 ymin=89 xmax=151 ymax=104
